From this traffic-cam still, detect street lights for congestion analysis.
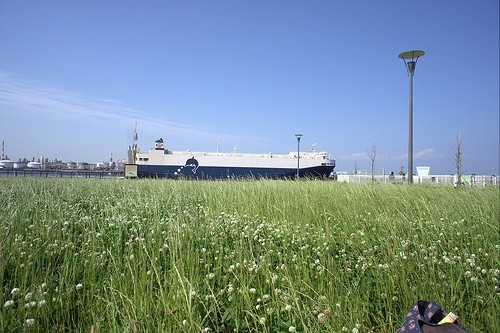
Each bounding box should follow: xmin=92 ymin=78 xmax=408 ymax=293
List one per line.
xmin=295 ymin=134 xmax=303 ymax=180
xmin=399 ymin=49 xmax=425 ymax=187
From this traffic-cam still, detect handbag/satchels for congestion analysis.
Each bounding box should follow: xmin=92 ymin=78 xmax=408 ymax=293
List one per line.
xmin=393 ymin=299 xmax=471 ymax=333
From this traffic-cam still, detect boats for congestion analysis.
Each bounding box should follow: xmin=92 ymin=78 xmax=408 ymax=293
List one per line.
xmin=129 ymin=136 xmax=337 ymax=182
